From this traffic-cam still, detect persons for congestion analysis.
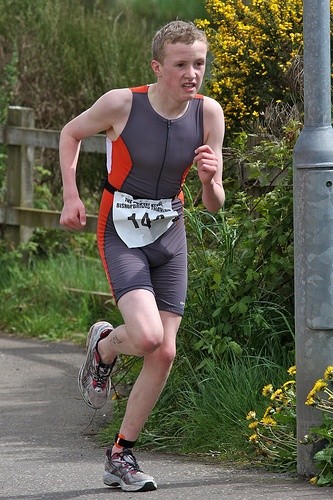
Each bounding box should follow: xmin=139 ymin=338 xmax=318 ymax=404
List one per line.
xmin=57 ymin=16 xmax=229 ymax=495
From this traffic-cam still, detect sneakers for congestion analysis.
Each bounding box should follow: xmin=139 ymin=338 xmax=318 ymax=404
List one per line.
xmin=76 ymin=321 xmax=114 ymax=410
xmin=102 ymin=448 xmax=158 ymax=492
